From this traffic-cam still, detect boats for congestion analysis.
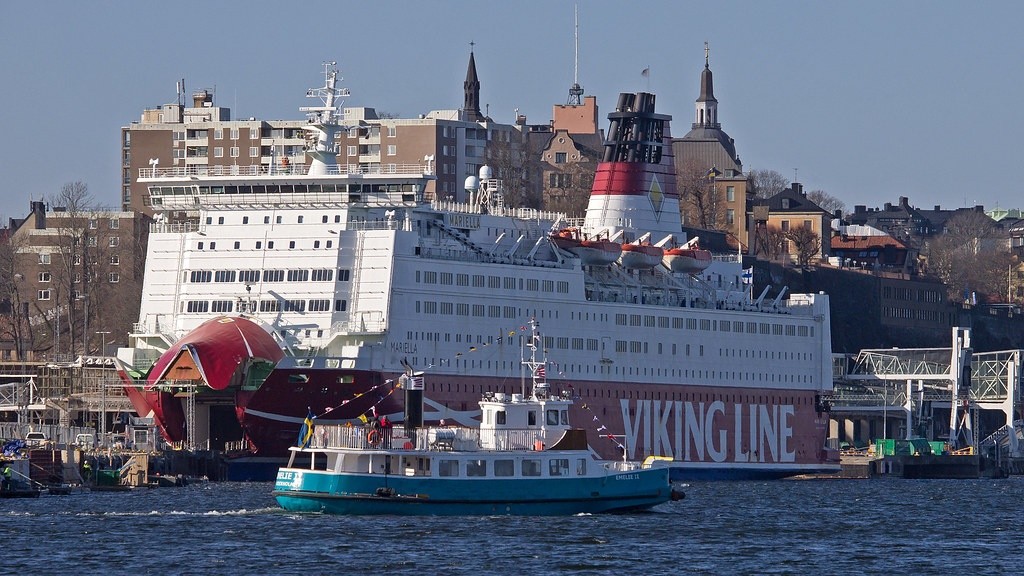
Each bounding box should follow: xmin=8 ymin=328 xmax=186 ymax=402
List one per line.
xmin=663 ymin=236 xmax=714 ymax=277
xmin=0 ymin=457 xmax=75 ymax=498
xmin=546 ymin=226 xmax=623 ymax=268
xmin=273 ymin=321 xmax=688 ymax=517
xmin=614 ymin=230 xmax=664 ymax=271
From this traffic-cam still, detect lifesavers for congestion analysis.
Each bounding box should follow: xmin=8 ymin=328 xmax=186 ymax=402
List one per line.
xmin=367 ymin=430 xmax=383 ymax=445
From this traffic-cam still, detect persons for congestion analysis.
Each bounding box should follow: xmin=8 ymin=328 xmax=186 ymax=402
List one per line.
xmin=438 ymin=419 xmax=448 ymax=429
xmin=82 ymin=460 xmax=93 ymax=483
xmin=339 ymin=414 xmax=392 ymax=449
xmin=2 ymin=463 xmax=12 ymax=486
xmin=114 ymin=437 xmax=137 ymax=451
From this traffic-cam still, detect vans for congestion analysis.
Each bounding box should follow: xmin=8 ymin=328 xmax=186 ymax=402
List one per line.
xmin=75 ymin=433 xmax=93 ymax=449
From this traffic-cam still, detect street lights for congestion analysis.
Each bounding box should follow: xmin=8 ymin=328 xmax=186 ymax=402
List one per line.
xmin=96 ymin=331 xmax=111 ymax=447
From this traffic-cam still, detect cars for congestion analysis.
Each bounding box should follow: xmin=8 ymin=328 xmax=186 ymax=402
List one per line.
xmin=25 ymin=431 xmax=50 ymax=449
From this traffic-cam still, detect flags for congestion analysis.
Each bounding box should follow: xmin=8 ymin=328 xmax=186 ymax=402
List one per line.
xmin=703 ymin=167 xmax=722 ymax=182
xmin=741 ymin=268 xmax=753 ymax=284
xmin=298 ymin=406 xmax=314 ymax=451
xmin=537 ymin=367 xmax=546 ymax=379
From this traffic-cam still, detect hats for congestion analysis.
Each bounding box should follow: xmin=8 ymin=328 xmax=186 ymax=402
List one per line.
xmin=374 ymin=413 xmax=379 ymax=419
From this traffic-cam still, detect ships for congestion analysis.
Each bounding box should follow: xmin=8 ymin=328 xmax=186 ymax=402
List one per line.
xmin=109 ymin=59 xmax=845 ymax=482
